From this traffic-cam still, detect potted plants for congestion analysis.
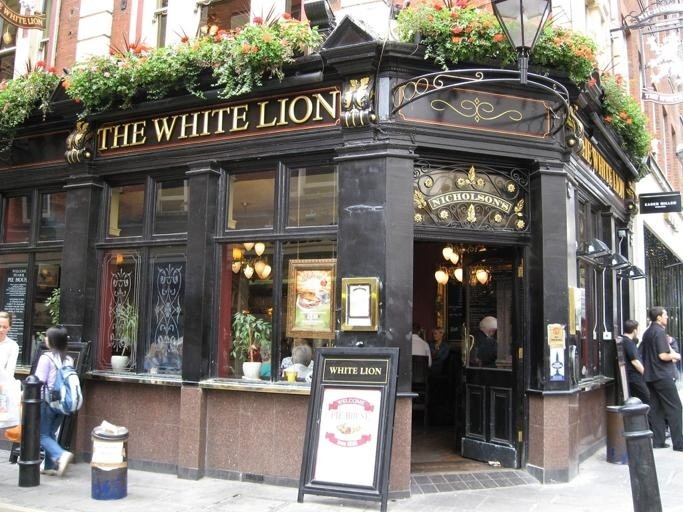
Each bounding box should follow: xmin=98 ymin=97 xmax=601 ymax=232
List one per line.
xmin=110 ymin=303 xmax=137 ymax=368
xmin=230 ymin=312 xmax=272 ymax=381
xmin=35 ymin=287 xmax=60 ymax=349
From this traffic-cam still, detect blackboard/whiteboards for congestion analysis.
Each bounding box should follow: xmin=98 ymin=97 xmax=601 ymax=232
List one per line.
xmin=0 ymin=266 xmax=37 ymax=366
xmin=444 ymin=271 xmax=526 ymax=341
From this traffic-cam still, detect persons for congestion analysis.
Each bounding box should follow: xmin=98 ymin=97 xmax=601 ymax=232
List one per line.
xmin=0 ymin=311 xmax=20 ymax=426
xmin=34 ymin=323 xmax=74 ymax=477
xmin=411 ymin=316 xmax=497 ymax=421
xmin=622 ymin=306 xmax=683 ymax=451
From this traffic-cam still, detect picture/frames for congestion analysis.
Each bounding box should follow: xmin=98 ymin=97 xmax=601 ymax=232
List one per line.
xmin=286 ymin=259 xmax=335 ymax=341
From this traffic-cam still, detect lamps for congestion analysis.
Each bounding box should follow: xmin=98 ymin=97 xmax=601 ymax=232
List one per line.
xmin=233 ymin=242 xmax=272 ymax=280
xmin=116 ymin=254 xmax=122 ymax=265
xmin=435 ymin=242 xmax=492 ymax=286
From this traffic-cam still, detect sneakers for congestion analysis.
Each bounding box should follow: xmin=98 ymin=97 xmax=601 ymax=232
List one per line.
xmin=57 ymin=451 xmax=74 ymax=477
xmin=40 ymin=469 xmax=57 ymax=476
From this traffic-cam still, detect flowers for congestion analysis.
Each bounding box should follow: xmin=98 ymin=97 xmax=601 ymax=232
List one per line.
xmin=0 ymin=1 xmax=325 ymax=137
xmin=395 ymin=0 xmax=655 ymax=166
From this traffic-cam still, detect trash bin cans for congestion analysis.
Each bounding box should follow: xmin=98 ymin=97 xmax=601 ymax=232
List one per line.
xmin=605 ymin=406 xmax=628 ymax=464
xmin=90 ymin=427 xmax=129 ymax=500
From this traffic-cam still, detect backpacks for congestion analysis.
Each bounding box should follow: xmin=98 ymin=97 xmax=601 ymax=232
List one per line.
xmin=44 ymin=352 xmax=84 ymax=417
